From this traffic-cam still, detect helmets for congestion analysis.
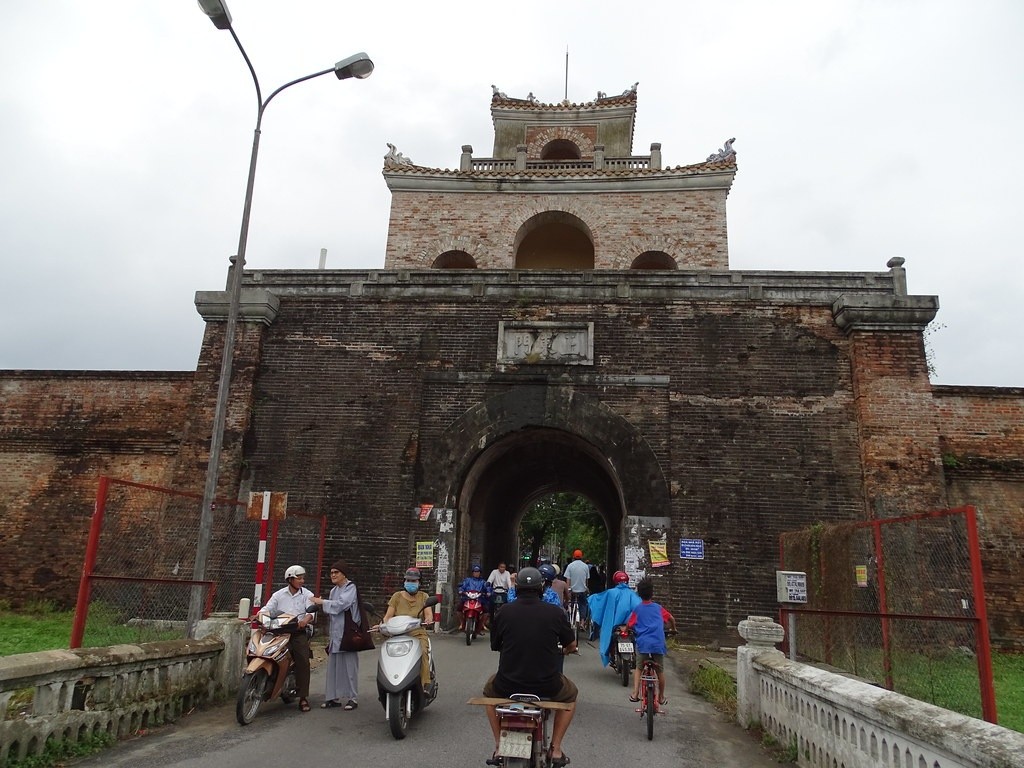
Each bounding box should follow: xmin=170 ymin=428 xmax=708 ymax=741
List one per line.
xmin=285 ymin=565 xmax=305 ymax=579
xmin=404 ymin=568 xmax=421 ymax=580
xmin=613 ymin=571 xmax=629 ymax=584
xmin=537 ymin=564 xmax=556 ymax=578
xmin=471 ymin=565 xmax=482 ymax=574
xmin=552 ymin=564 xmax=560 ymax=576
xmin=515 ymin=567 xmax=542 ymax=593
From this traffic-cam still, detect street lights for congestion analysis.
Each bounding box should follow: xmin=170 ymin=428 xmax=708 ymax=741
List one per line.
xmin=186 ymin=0 xmax=374 ymax=634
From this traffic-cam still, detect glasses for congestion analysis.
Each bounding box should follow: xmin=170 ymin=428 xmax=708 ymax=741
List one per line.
xmin=330 ymin=571 xmax=339 ymax=575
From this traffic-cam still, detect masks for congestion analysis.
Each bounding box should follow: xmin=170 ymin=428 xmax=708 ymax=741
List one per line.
xmin=404 ymin=582 xmax=419 ymax=592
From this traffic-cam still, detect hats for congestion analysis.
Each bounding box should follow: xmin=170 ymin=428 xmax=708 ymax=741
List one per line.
xmin=330 ymin=559 xmax=347 ymax=575
xmin=573 ymin=550 xmax=582 ymax=559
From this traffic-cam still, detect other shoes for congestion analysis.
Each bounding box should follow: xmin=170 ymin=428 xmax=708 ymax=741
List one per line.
xmin=424 ymin=690 xmax=430 ymax=695
xmin=580 ymin=624 xmax=587 ymax=631
xmin=610 ymin=655 xmax=615 ymax=662
xmin=477 ymin=631 xmax=484 ymax=636
xmin=459 ymin=624 xmax=463 ymax=630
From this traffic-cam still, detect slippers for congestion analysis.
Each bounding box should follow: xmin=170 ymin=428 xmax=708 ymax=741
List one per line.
xmin=344 ymin=700 xmax=358 ymax=710
xmin=629 ymin=696 xmax=638 ymax=702
xmin=298 ymin=699 xmax=312 ymax=712
xmin=552 ymin=750 xmax=570 ymax=765
xmin=320 ymin=700 xmax=342 ymax=708
xmin=659 ymin=697 xmax=666 ymax=705
xmin=492 ymin=750 xmax=504 ymax=764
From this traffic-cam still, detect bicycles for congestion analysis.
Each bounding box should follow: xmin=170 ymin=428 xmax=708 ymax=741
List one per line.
xmin=625 ymin=629 xmax=679 ymax=740
xmin=566 ymin=589 xmax=581 ymax=647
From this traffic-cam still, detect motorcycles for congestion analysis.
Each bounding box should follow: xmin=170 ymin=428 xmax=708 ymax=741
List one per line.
xmin=609 ymin=625 xmax=638 ymax=687
xmin=485 ymin=647 xmax=580 ymax=768
xmin=236 ymin=605 xmax=320 ymax=725
xmin=457 ymin=581 xmax=508 ymax=645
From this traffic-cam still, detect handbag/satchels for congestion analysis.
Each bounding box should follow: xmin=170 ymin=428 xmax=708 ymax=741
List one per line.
xmin=339 ymin=610 xmax=376 ymax=653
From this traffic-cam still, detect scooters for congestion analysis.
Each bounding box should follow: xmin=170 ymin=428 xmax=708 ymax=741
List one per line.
xmin=360 ymin=595 xmax=440 ymax=740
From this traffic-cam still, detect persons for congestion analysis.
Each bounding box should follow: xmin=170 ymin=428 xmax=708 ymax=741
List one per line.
xmin=457 ymin=565 xmax=492 ymax=636
xmin=598 ymin=565 xmax=606 ymax=591
xmin=623 ymin=578 xmax=678 ymax=705
xmin=588 ymin=567 xmax=603 ymax=595
xmin=372 ymin=567 xmax=433 ymax=694
xmin=563 ymin=550 xmax=590 ymax=632
xmin=309 ymin=559 xmax=362 ymax=710
xmin=507 ymin=563 xmax=563 ymax=608
xmin=483 ymin=566 xmax=579 ymax=768
xmin=487 ymin=562 xmax=511 ymax=600
xmin=249 ymin=565 xmax=317 ymax=712
xmin=586 ymin=570 xmax=642 ymax=667
xmin=562 ymin=558 xmax=572 ymax=576
xmin=584 ymin=559 xmax=592 ymax=570
